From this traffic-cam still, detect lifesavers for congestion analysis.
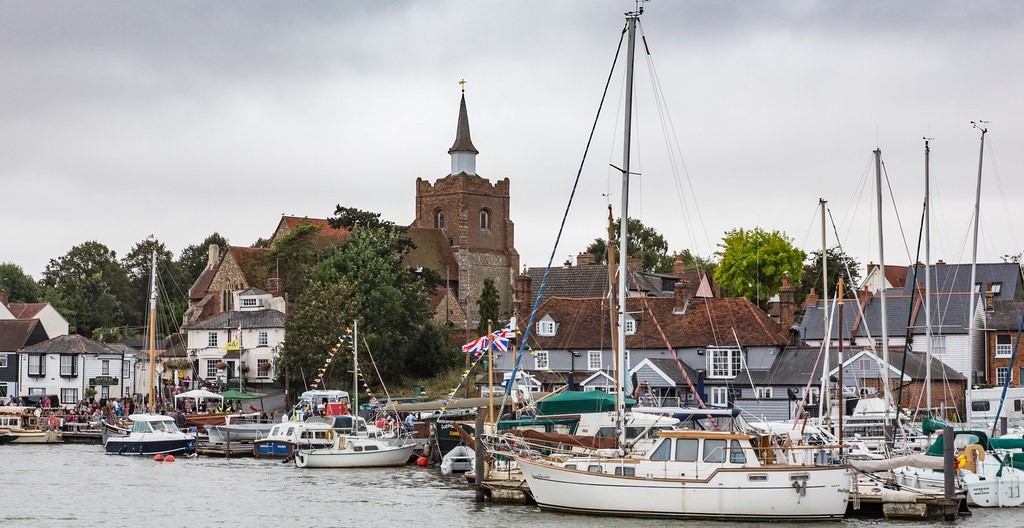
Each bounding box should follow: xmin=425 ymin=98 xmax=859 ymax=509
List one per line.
xmin=965 ymin=445 xmax=985 ymax=463
xmin=500 ymin=432 xmax=516 ymax=451
xmin=375 ymin=418 xmax=389 ymax=430
xmin=46 ymin=418 xmax=57 ymax=428
xmin=38 ymin=417 xmax=47 ymax=427
xmin=325 ymin=429 xmax=334 ymax=440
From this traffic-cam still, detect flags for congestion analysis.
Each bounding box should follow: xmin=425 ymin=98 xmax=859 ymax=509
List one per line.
xmin=223 ymin=338 xmax=240 ymax=350
xmin=462 ymin=323 xmax=510 ymax=352
xmin=233 ymin=324 xmax=240 ymax=336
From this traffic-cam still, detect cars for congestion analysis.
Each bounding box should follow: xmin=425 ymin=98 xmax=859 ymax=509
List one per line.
xmin=204 ymin=377 xmax=223 ymax=393
xmin=359 ymin=398 xmax=419 ymax=415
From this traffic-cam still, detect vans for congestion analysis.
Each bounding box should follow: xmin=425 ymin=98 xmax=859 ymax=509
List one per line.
xmin=6 ymin=395 xmax=60 ymax=408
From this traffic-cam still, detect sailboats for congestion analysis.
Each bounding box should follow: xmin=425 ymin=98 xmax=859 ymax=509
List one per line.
xmin=817 ymin=121 xmax=1024 ymax=508
xmin=505 ymin=8 xmax=851 ymax=522
xmin=294 ymin=320 xmax=419 ymax=467
xmin=108 ymin=244 xmax=240 ymax=444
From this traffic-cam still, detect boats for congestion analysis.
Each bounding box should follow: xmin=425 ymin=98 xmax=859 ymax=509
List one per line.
xmin=254 ymin=414 xmax=394 ymax=457
xmin=104 ymin=414 xmax=196 ymax=456
xmin=99 ymin=419 xmax=198 ymax=446
xmin=0 ymin=406 xmax=65 ymax=444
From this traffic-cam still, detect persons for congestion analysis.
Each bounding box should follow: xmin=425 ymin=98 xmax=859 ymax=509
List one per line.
xmin=404 ymin=412 xmax=418 ymax=431
xmin=9 ymin=392 xmax=274 ymax=431
